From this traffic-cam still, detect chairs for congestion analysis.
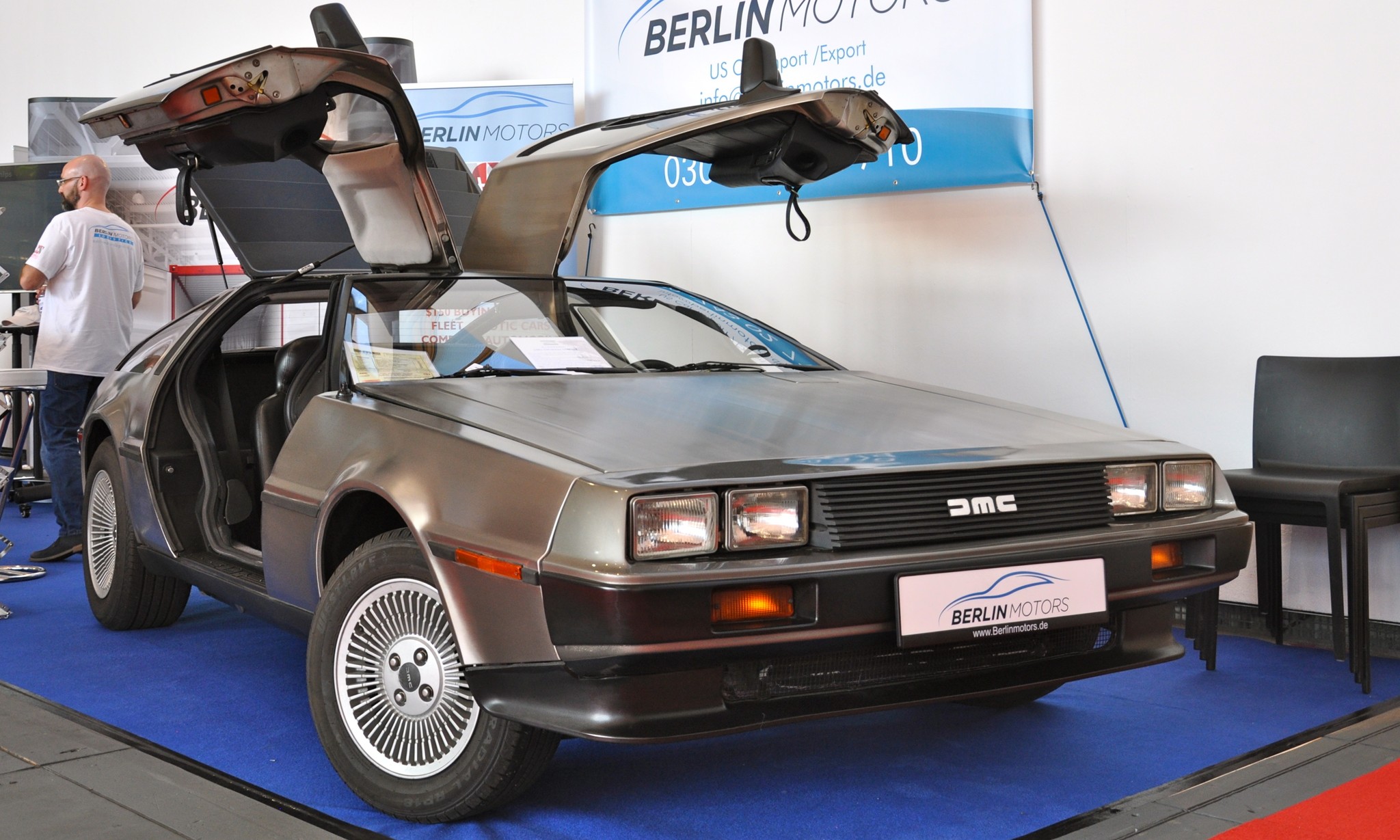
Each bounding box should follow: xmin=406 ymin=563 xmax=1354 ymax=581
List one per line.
xmin=254 ymin=335 xmax=325 ymax=485
xmin=1184 ymin=355 xmax=1400 ymax=695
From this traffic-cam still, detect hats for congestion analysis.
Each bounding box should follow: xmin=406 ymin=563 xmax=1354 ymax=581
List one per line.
xmin=2 ymin=304 xmax=41 ymax=326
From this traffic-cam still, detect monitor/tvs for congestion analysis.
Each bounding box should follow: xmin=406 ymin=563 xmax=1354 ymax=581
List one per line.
xmin=0 ymin=163 xmax=68 ymax=293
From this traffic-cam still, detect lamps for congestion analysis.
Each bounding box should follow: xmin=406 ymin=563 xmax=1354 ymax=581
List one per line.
xmin=171 ymin=228 xmax=180 ymax=240
xmin=132 ymin=165 xmax=145 ymax=205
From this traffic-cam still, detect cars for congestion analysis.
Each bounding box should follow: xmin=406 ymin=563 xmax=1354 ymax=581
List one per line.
xmin=69 ymin=46 xmax=1256 ymax=826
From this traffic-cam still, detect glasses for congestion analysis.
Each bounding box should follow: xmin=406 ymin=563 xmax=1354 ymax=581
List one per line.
xmin=56 ymin=176 xmax=87 ymax=187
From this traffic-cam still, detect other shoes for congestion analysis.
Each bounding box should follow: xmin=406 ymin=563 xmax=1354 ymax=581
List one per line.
xmin=30 ymin=534 xmax=83 ymax=561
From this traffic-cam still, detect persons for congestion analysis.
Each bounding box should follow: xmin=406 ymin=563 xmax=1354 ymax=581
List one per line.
xmin=19 ymin=155 xmax=144 ymax=561
